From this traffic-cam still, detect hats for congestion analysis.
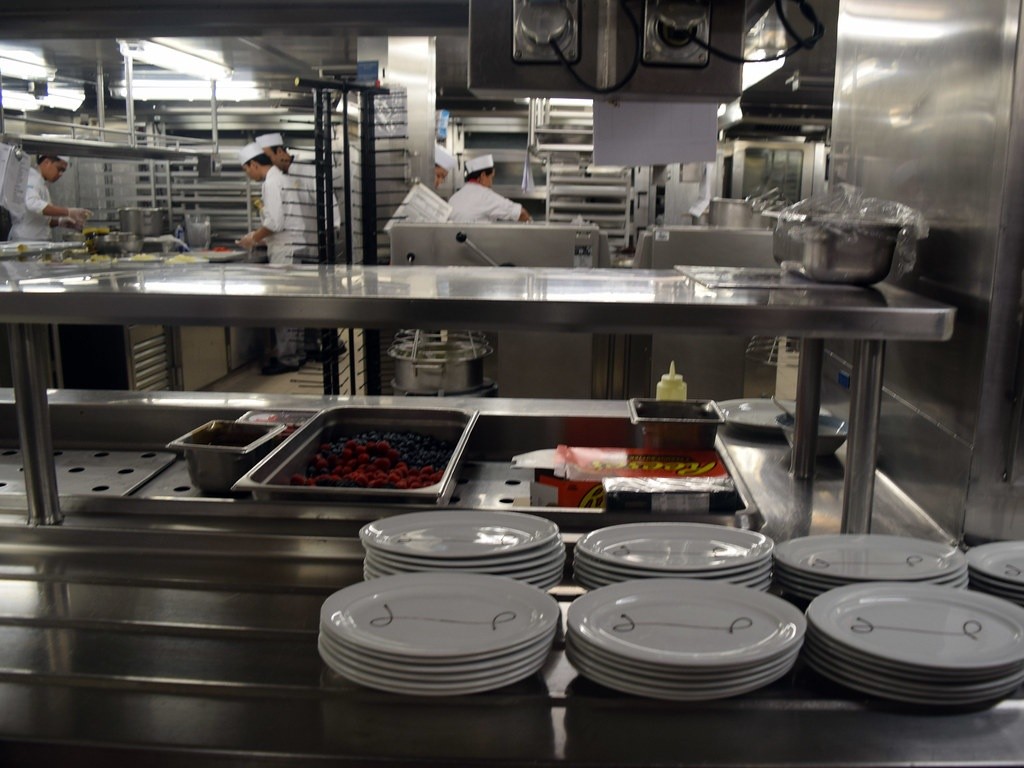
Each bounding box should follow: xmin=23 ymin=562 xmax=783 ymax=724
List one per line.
xmin=465 ymin=153 xmax=494 ymax=174
xmin=57 ymin=155 xmax=71 ymax=162
xmin=238 ymin=141 xmax=265 ymax=166
xmin=254 ymin=132 xmax=284 ymax=148
xmin=435 ymin=143 xmax=455 ymax=173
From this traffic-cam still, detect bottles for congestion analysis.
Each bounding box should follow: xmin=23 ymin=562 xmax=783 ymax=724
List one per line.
xmin=655 ymin=361 xmax=688 ymax=400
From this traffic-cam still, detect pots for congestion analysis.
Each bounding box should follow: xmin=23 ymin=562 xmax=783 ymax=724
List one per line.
xmin=118 ymin=207 xmax=165 ymax=237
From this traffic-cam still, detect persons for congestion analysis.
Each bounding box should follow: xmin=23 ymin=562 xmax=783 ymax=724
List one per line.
xmin=235 ymin=131 xmax=346 ymax=376
xmin=9 ymin=153 xmax=94 ymax=243
xmin=434 ymin=144 xmax=532 ymax=224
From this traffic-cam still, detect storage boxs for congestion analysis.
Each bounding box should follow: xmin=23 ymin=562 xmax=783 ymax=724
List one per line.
xmin=510 ymin=445 xmax=735 ymax=508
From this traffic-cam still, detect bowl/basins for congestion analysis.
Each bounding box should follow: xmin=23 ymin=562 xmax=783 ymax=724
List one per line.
xmin=93 ymin=234 xmax=145 ymax=256
xmin=761 ymin=209 xmax=914 ymax=284
xmin=775 ymin=412 xmax=847 ymax=456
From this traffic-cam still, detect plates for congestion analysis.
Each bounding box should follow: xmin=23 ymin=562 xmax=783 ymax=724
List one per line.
xmin=717 ymin=398 xmax=832 ymax=437
xmin=314 ymin=509 xmax=1024 ymax=710
